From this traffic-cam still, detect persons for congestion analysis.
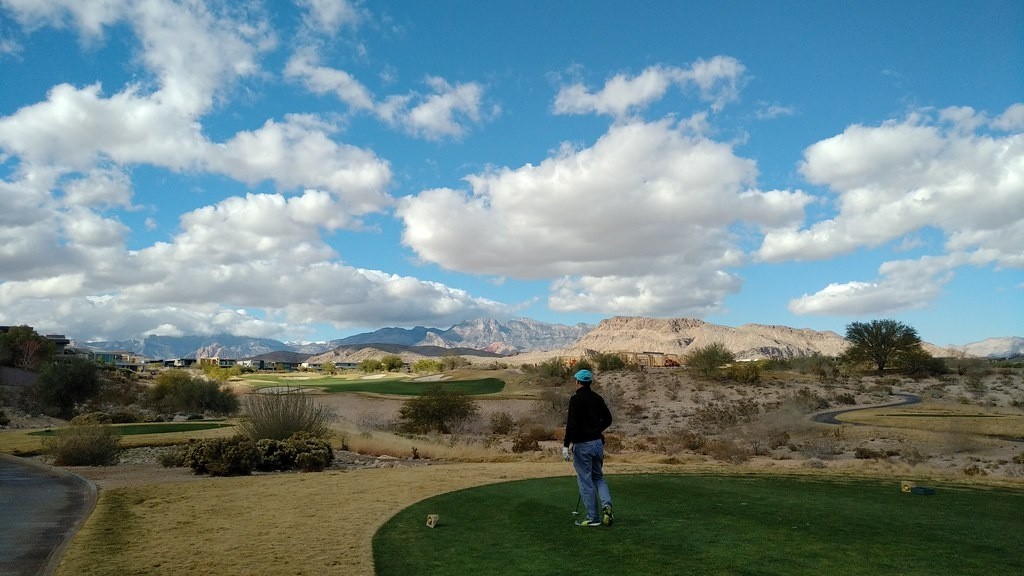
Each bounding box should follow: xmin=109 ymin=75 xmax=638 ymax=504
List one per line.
xmin=562 ymin=369 xmax=614 ymax=526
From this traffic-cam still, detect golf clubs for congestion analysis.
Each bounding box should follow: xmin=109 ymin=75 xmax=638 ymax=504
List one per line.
xmin=572 ymin=495 xmax=582 ymax=515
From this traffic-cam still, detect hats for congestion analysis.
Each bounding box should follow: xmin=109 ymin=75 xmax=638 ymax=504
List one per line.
xmin=573 ymin=369 xmax=593 ymax=381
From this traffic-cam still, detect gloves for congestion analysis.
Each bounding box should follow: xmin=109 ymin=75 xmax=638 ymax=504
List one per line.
xmin=562 ymin=447 xmax=570 ymax=462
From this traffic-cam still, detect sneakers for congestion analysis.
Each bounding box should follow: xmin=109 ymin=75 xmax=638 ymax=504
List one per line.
xmin=602 ymin=504 xmax=614 ymax=527
xmin=574 ymin=518 xmax=601 ymax=527
